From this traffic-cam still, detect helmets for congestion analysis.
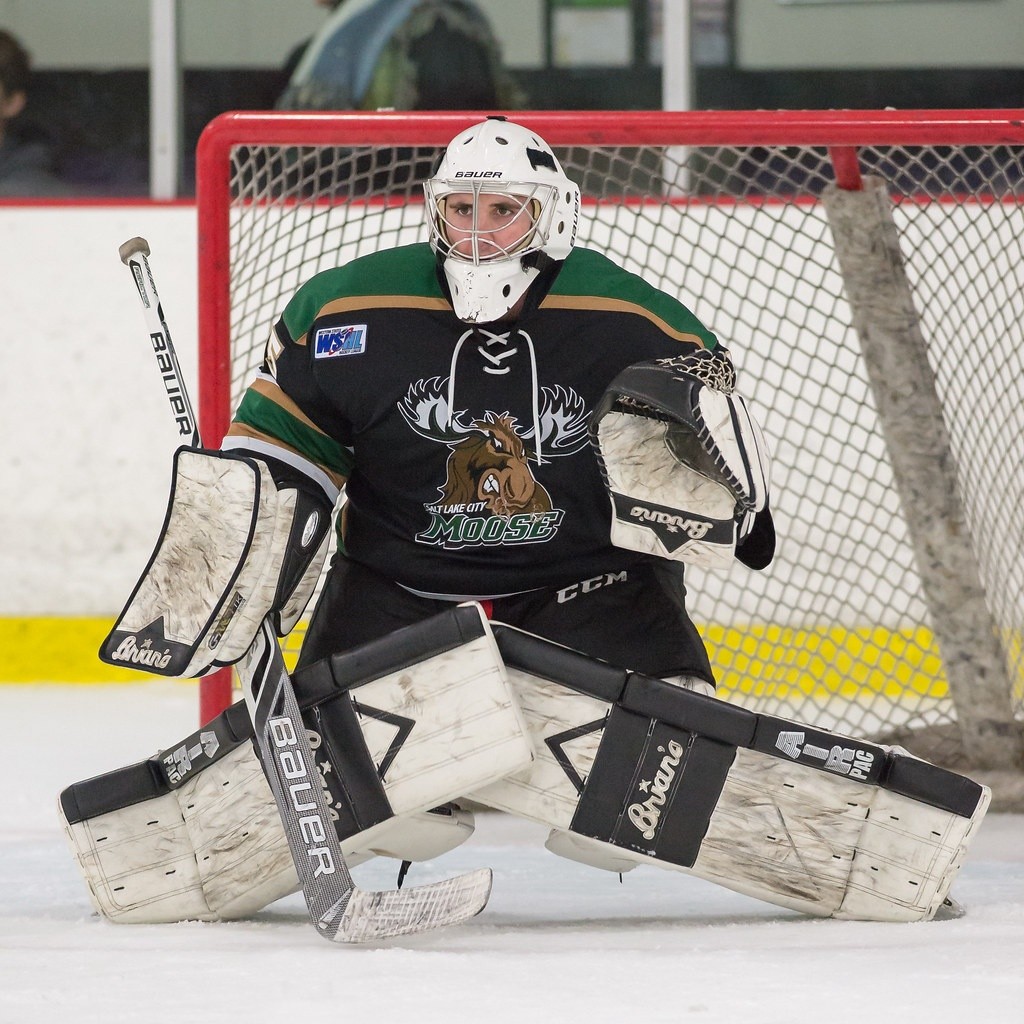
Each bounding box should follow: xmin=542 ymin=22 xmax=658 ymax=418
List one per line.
xmin=422 ymin=115 xmax=581 ymax=325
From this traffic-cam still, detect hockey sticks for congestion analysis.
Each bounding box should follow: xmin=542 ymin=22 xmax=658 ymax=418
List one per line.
xmin=118 ymin=237 xmax=495 ymax=946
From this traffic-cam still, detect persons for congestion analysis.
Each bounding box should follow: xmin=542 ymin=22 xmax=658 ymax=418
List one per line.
xmin=1 ymin=1 xmax=527 ymax=194
xmin=58 ymin=119 xmax=991 ymax=929
